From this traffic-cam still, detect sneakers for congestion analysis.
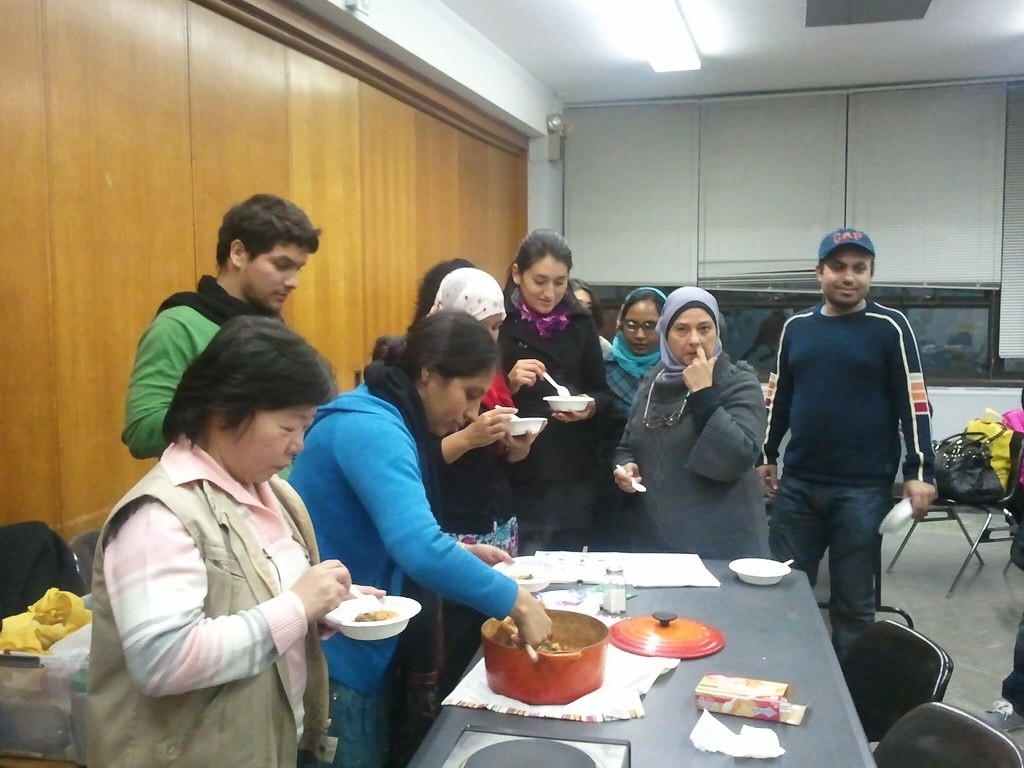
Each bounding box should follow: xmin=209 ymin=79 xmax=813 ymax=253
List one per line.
xmin=975 ymin=699 xmax=1024 ymax=733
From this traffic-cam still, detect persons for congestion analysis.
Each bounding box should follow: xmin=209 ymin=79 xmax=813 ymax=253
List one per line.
xmin=981 ymin=389 xmax=1024 ymax=735
xmin=287 ymin=309 xmax=553 ymax=768
xmin=754 ymin=228 xmax=938 ymax=656
xmin=388 ymin=258 xmax=548 ymax=766
xmin=495 ymin=229 xmax=612 ymax=556
xmin=609 ymin=285 xmax=772 ymax=559
xmin=122 ymin=192 xmax=323 ymax=478
xmin=80 ymin=316 xmax=386 ymax=768
xmin=568 ymin=278 xmax=611 ymax=357
xmin=603 ymin=288 xmax=668 ymax=442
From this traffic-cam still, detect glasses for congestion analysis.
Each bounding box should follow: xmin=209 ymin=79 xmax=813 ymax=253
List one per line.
xmin=626 ymin=320 xmax=657 ymax=333
xmin=643 ymin=398 xmax=686 ymax=430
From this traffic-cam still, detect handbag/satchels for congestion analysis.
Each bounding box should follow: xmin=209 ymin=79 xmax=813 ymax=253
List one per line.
xmin=930 ymin=408 xmax=1014 ymax=506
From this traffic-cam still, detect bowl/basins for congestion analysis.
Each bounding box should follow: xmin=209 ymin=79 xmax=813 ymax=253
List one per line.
xmin=507 ymin=417 xmax=547 ymax=436
xmin=492 ymin=556 xmax=557 ymax=592
xmin=543 ymin=396 xmax=594 ymax=412
xmin=323 ymin=595 xmax=421 ymax=640
xmin=728 ymin=558 xmax=792 ymax=586
xmin=878 ymin=496 xmax=913 ymax=533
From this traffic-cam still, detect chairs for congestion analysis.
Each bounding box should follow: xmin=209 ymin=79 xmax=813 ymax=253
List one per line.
xmin=68 ymin=528 xmax=100 ymax=594
xmin=885 ymin=431 xmax=1024 ymax=598
xmin=1 ymin=521 xmax=85 ymax=620
xmin=872 ymin=701 xmax=1024 ymax=768
xmin=840 ymin=620 xmax=955 ymax=741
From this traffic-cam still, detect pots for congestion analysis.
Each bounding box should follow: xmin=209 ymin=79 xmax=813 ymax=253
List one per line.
xmin=481 ymin=609 xmax=609 ymax=706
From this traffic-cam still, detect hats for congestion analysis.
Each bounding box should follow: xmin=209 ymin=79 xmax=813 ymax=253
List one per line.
xmin=819 ymin=228 xmax=875 ymax=263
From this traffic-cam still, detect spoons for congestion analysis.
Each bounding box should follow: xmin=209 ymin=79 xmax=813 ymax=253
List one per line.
xmin=543 ymin=372 xmax=570 ymax=395
xmin=350 ymin=584 xmax=382 ymax=610
xmin=616 ymin=465 xmax=647 ymax=492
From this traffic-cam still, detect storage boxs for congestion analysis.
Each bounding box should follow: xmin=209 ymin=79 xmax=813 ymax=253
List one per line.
xmin=695 ymin=672 xmax=812 ymax=726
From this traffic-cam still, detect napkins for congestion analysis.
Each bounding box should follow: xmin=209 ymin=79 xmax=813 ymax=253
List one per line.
xmin=690 ymin=709 xmax=785 ymax=759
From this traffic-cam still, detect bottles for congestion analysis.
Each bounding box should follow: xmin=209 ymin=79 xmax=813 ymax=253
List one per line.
xmin=601 ymin=565 xmax=626 ymax=614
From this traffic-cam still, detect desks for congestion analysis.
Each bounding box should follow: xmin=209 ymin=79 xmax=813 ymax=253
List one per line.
xmin=408 ymin=560 xmax=880 ymax=768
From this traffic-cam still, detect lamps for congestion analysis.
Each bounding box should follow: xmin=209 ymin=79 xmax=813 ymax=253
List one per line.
xmin=547 ymin=114 xmax=574 ymax=164
